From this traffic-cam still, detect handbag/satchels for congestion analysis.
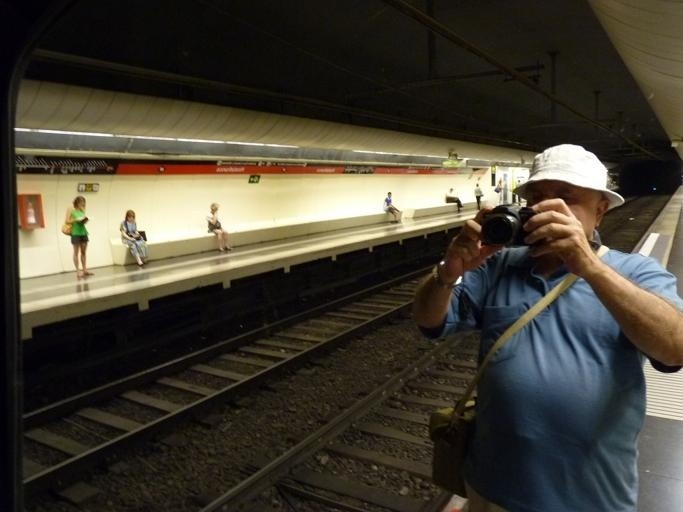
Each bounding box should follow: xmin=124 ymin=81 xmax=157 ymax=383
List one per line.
xmin=429 ymin=399 xmax=477 ymax=498
xmin=208 ymin=221 xmax=220 ymax=230
xmin=62 ymin=224 xmax=71 ymax=235
xmin=136 ymin=231 xmax=146 ymax=241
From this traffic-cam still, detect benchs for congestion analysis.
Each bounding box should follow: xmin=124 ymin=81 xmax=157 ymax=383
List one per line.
xmin=110 ymin=207 xmax=403 ymax=265
xmin=404 ymin=200 xmax=489 ymax=219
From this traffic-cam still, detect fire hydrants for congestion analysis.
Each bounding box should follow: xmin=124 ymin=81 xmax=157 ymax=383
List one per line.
xmin=19 ymin=194 xmax=43 ymax=228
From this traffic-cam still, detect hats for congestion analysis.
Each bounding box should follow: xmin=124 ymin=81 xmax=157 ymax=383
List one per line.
xmin=513 ymin=144 xmax=625 ymax=211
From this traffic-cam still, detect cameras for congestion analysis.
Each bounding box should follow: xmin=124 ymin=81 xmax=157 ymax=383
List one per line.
xmin=480 ymin=204 xmax=545 ymax=247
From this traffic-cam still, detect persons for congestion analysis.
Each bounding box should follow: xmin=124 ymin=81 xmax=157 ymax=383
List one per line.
xmin=494 ymin=177 xmax=504 ymax=204
xmin=410 ymin=144 xmax=682 ymax=511
xmin=514 ymin=180 xmax=522 ymax=206
xmin=118 ymin=210 xmax=149 ymax=266
xmin=474 ymin=183 xmax=484 ymax=210
xmin=64 ymin=196 xmax=93 ymax=278
xmin=383 ymin=192 xmax=400 ymax=222
xmin=205 ymin=203 xmax=232 ymax=252
xmin=75 ymin=277 xmax=90 ymax=301
xmin=445 ymin=188 xmax=464 ymax=213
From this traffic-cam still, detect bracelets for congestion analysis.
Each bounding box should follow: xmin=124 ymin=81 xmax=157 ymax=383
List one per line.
xmin=431 ymin=262 xmax=463 ymax=289
